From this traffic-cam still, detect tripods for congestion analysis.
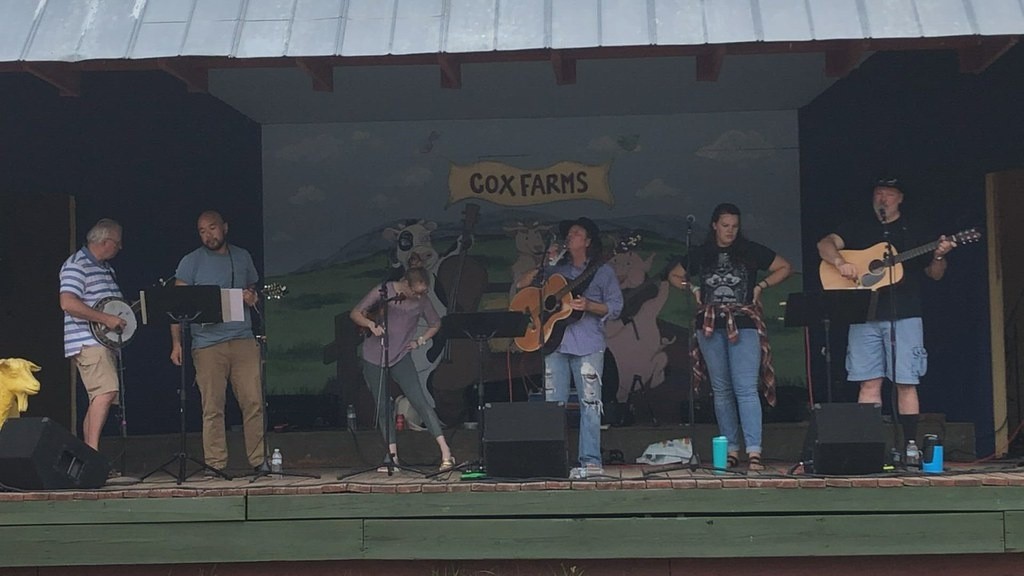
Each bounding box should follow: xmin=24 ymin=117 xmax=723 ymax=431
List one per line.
xmin=140 ymin=282 xmax=323 ymax=487
xmin=425 ymin=310 xmax=531 ymax=479
xmin=337 ymin=268 xmax=429 ymax=481
xmin=640 ymin=223 xmax=746 ymax=477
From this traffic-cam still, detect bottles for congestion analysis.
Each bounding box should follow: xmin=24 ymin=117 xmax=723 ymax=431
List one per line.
xmin=396 ymin=414 xmax=404 ymax=432
xmin=347 ymin=405 xmax=356 ymax=432
xmin=272 ymin=449 xmax=282 ymax=480
xmin=923 ymin=432 xmax=939 ymax=463
xmin=712 ymin=435 xmax=728 ymax=475
xmin=907 ymin=440 xmax=919 ymax=473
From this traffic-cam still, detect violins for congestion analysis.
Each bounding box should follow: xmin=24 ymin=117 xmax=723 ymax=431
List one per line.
xmin=351 ymin=290 xmax=408 ymax=338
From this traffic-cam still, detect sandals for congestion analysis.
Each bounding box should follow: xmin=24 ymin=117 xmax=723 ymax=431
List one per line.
xmin=747 ymin=455 xmax=765 ymax=471
xmin=727 ymin=455 xmax=740 ymax=468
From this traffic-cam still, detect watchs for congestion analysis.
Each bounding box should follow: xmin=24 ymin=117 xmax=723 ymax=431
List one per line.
xmin=934 ymin=255 xmax=945 ymax=261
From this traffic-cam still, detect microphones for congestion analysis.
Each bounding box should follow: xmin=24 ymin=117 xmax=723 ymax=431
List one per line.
xmin=545 ymin=233 xmax=556 ymax=250
xmin=388 ymin=254 xmax=401 ymax=267
xmin=878 ymin=206 xmax=886 ymax=218
xmin=687 ymin=215 xmax=695 ymax=223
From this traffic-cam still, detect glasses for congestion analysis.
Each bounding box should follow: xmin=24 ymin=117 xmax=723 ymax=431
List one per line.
xmin=408 ymin=281 xmax=428 ymax=298
xmin=104 ymin=236 xmax=123 ymax=248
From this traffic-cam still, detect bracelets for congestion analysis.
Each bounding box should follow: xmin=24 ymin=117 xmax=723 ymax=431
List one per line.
xmin=756 ymin=279 xmax=769 ymax=290
xmin=416 ymin=336 xmax=426 ymax=347
xmin=692 ymin=286 xmax=700 ymax=294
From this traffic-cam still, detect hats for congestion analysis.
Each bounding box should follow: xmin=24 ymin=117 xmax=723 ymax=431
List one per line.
xmin=558 ymin=217 xmax=600 ymax=241
xmin=872 ymin=173 xmax=905 ymax=193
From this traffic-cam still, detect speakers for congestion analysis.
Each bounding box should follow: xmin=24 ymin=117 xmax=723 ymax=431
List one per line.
xmin=800 ymin=403 xmax=886 ymax=475
xmin=0 ymin=418 xmax=110 ymax=493
xmin=483 ymin=401 xmax=571 ymax=481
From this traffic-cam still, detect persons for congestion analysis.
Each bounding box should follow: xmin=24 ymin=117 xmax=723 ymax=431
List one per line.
xmin=516 ymin=216 xmax=624 ymax=477
xmin=59 ymin=218 xmax=150 ymax=479
xmin=170 ymin=210 xmax=272 ymax=480
xmin=349 ymin=266 xmax=455 ymax=472
xmin=669 ymin=201 xmax=791 ymax=470
xmin=817 ymin=179 xmax=958 ymax=468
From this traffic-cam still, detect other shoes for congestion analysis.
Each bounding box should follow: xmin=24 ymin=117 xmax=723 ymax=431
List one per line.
xmin=255 ymin=463 xmax=270 ymax=475
xmin=108 ymin=468 xmax=122 ymax=478
xmin=204 ymin=467 xmax=220 ymax=477
xmin=378 ymin=466 xmax=400 ymax=473
xmin=439 ymin=456 xmax=456 ymax=470
xmin=583 ymin=463 xmax=603 ymax=474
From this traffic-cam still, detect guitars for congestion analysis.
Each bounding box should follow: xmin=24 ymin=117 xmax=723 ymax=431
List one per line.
xmin=88 ymin=272 xmax=176 ymax=351
xmin=819 ymin=225 xmax=982 ymax=291
xmin=509 ymin=249 xmax=611 ymax=356
xmin=244 ymin=281 xmax=289 ymax=303
